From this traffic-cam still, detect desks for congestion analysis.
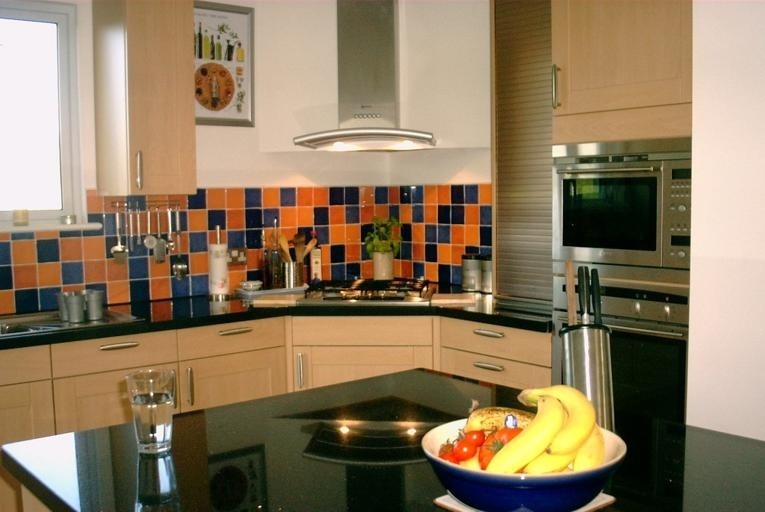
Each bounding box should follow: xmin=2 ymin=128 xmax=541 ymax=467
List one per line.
xmin=3 ymin=366 xmax=764 ymax=512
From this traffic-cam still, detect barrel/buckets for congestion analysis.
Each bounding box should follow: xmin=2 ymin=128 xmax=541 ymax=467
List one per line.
xmin=54 ymin=289 xmax=105 ymax=322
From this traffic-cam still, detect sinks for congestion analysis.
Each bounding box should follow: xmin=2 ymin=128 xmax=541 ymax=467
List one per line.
xmin=0 ymin=324 xmax=58 ymax=337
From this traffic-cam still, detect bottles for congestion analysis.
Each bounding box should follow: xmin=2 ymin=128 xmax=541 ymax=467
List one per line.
xmin=265 ymin=248 xmax=284 ymax=286
xmin=462 ymin=293 xmax=494 ymax=315
xmin=460 ymin=253 xmax=492 ymax=295
xmin=306 ymin=232 xmax=324 ymax=283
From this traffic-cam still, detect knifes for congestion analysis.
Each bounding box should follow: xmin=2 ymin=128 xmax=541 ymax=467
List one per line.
xmin=563 ymin=262 xmax=604 ymax=325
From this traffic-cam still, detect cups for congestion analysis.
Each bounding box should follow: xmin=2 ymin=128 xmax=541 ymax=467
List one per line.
xmin=123 ymin=367 xmax=176 ymax=458
xmin=12 ymin=209 xmax=29 ymax=226
xmin=135 ymin=452 xmax=183 ymax=512
xmin=279 ymin=261 xmax=305 ymax=289
xmin=61 ymin=215 xmax=77 ymax=224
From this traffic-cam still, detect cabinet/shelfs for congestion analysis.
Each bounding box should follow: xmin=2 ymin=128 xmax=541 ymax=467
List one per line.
xmin=1 ymin=345 xmax=57 ymax=511
xmin=177 ymin=316 xmax=286 ymax=416
xmin=49 ymin=329 xmax=180 ymax=434
xmin=92 ymin=1 xmax=197 ymax=197
xmin=549 ymin=0 xmax=693 ymax=145
xmin=440 ymin=316 xmax=550 ymax=392
xmin=292 ymin=314 xmax=432 ymax=394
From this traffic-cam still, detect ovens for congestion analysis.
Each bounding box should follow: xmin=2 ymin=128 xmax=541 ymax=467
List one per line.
xmin=550 ymin=136 xmax=693 ymax=427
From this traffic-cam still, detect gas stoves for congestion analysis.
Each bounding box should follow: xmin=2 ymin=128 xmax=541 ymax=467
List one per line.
xmin=305 ymin=276 xmax=429 ymax=299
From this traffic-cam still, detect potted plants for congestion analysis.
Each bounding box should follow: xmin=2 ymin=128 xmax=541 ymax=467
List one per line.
xmin=365 ymin=215 xmax=403 ymax=280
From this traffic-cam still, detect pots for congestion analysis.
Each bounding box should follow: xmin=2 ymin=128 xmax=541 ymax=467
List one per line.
xmin=55 ymin=288 xmax=105 ymax=323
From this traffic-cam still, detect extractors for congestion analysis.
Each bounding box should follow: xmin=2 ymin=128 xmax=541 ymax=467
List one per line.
xmin=293 ymin=2 xmax=440 ymax=153
xmin=300 ymin=421 xmax=430 ymax=510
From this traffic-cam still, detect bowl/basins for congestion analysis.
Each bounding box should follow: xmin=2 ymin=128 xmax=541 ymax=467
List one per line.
xmin=240 ymin=281 xmax=262 ymax=291
xmin=422 ymin=416 xmax=627 ymax=511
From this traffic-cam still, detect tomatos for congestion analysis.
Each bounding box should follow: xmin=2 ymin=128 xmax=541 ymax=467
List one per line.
xmin=440 ymin=429 xmax=523 ymax=471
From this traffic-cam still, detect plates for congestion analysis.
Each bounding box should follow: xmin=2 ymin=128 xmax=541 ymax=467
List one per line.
xmin=432 ymin=493 xmax=617 ymax=512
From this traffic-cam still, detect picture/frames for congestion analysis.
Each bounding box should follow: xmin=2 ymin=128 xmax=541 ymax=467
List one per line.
xmin=193 ymin=1 xmax=254 ymax=127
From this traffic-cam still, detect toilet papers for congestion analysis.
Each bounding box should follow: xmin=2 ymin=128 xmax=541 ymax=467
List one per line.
xmin=208 ymin=244 xmax=229 ymax=295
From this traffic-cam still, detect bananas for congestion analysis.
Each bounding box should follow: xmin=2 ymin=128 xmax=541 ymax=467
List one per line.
xmin=485 ymin=385 xmax=603 ymax=474
xmin=464 ymin=404 xmax=536 ymax=428
